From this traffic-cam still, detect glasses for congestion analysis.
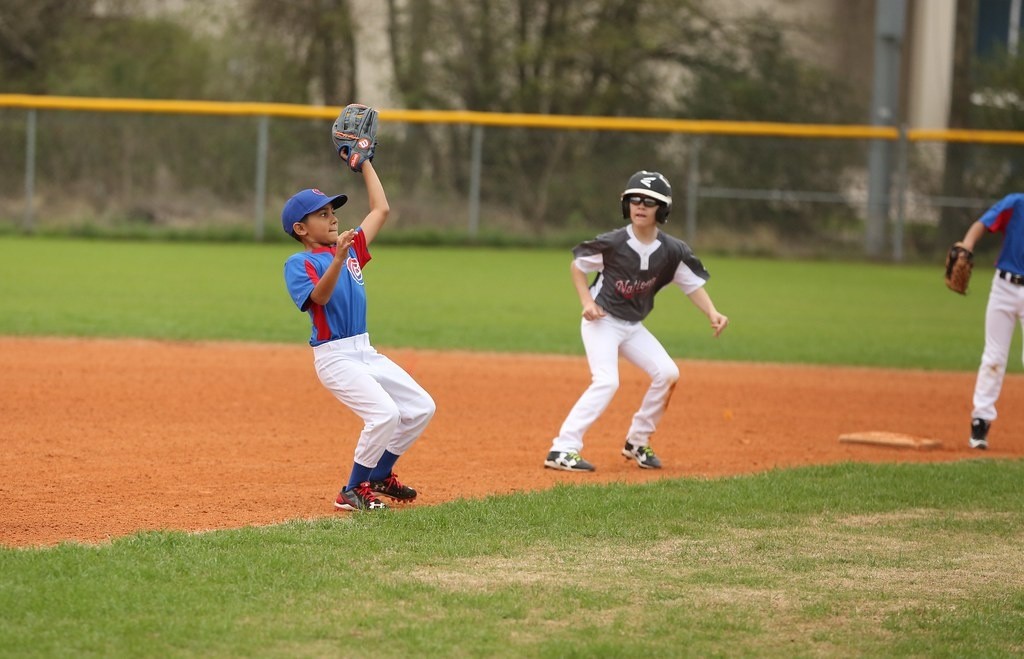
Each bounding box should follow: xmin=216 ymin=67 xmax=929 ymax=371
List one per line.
xmin=627 ymin=196 xmax=660 ymax=207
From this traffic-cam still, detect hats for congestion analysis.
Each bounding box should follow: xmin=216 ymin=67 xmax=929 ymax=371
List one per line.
xmin=282 ymin=189 xmax=348 ymax=235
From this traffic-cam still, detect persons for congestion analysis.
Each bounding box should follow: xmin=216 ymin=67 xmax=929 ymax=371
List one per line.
xmin=282 ymin=102 xmax=436 ymax=512
xmin=946 ymin=192 xmax=1024 ymax=448
xmin=544 ymin=170 xmax=729 ymax=473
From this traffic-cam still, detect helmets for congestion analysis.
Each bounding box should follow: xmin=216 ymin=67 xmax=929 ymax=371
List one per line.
xmin=621 ymin=171 xmax=672 ymax=224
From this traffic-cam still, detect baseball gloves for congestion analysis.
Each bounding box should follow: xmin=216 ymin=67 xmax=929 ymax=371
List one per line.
xmin=331 ymin=104 xmax=380 ymax=173
xmin=945 ymin=246 xmax=974 ymax=296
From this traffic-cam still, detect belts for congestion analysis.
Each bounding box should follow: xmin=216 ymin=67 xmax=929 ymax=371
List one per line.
xmin=999 ymin=271 xmax=1024 ymax=285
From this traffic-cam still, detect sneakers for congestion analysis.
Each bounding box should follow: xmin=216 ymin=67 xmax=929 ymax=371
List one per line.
xmin=334 ymin=480 xmax=389 ymax=515
xmin=370 ymin=469 xmax=417 ymax=503
xmin=969 ymin=418 xmax=991 ymax=449
xmin=544 ymin=451 xmax=595 ymax=472
xmin=622 ymin=442 xmax=661 ymax=470
